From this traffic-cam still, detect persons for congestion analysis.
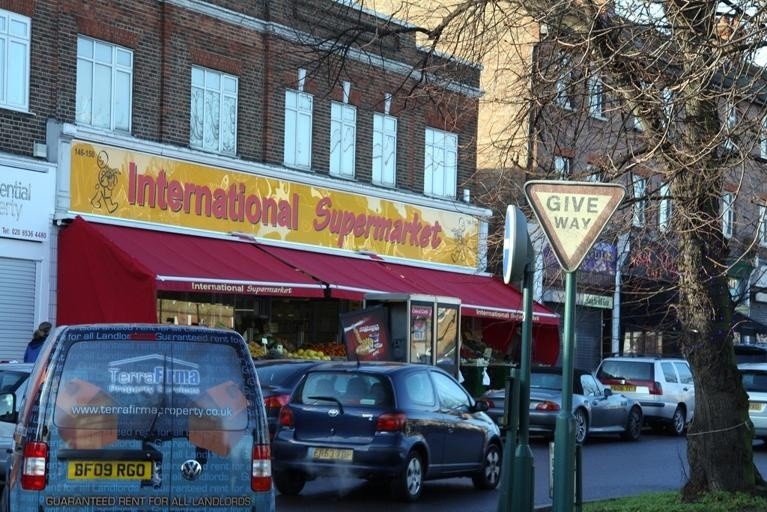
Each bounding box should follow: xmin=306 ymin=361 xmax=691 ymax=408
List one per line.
xmin=22 ymin=320 xmax=53 ymax=364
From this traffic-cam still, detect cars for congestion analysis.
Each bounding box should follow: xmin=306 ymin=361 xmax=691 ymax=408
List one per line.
xmin=0 ymin=317 xmax=767 ymax=511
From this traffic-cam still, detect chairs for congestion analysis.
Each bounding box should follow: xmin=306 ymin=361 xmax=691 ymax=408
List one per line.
xmin=314 ymin=376 xmax=384 ymax=404
